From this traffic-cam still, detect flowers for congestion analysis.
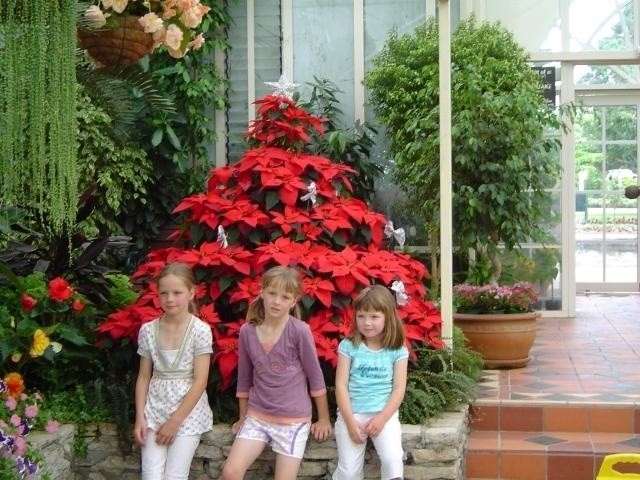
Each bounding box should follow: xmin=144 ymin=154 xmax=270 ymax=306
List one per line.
xmin=80 ymin=0 xmax=212 ymax=62
xmin=451 ymin=279 xmax=541 ymax=313
xmin=1 ymin=276 xmax=96 ymax=479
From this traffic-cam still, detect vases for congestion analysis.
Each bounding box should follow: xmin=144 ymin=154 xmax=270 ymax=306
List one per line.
xmin=451 ymin=314 xmax=539 ymax=370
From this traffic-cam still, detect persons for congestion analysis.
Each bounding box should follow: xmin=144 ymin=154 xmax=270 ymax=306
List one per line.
xmin=130 ymin=262 xmax=216 ymax=480
xmin=222 ymin=265 xmax=333 ymax=480
xmin=333 ymin=284 xmax=410 ymax=479
